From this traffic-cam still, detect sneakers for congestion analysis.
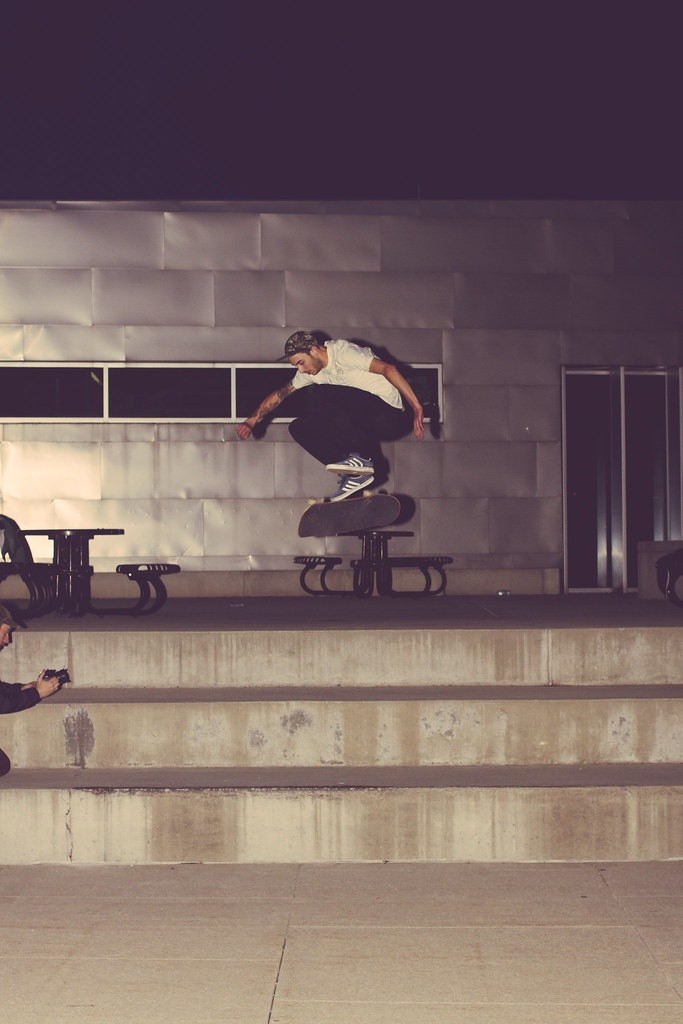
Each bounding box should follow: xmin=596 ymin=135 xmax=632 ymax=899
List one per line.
xmin=329 ymin=473 xmax=375 ymax=504
xmin=326 ymin=450 xmax=375 ymax=476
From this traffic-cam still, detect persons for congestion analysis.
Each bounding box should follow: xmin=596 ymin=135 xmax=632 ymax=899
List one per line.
xmin=235 ymin=331 xmax=425 ymax=502
xmin=0 ymin=605 xmax=60 ymax=776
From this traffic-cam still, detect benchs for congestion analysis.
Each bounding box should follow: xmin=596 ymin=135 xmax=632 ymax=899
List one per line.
xmin=386 ymin=552 xmax=452 ymax=569
xmin=116 ymin=559 xmax=180 ymax=577
xmin=0 ymin=561 xmax=52 ymax=576
xmin=295 ymin=554 xmax=342 ymax=570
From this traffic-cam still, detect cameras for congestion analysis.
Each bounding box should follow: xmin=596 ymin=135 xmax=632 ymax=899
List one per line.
xmin=42 ymin=669 xmax=71 ymax=690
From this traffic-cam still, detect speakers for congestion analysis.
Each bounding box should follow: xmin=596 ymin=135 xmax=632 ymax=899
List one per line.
xmin=639 ymin=538 xmax=683 ymax=601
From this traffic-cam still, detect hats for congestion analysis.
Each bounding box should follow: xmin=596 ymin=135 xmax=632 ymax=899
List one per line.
xmin=277 ymin=331 xmax=318 ymax=362
xmin=0 ymin=605 xmax=20 ymax=628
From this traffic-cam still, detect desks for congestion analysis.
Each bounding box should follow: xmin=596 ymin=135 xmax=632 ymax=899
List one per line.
xmin=338 ymin=529 xmax=413 ymax=602
xmin=16 ymin=527 xmax=126 ymax=616
